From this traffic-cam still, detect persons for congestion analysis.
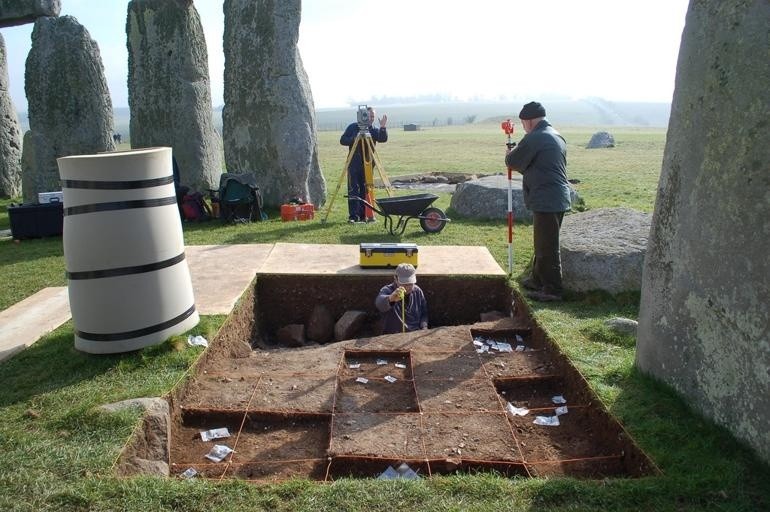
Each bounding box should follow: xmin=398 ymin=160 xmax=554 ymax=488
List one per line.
xmin=340 ymin=108 xmax=388 ymax=224
xmin=375 ymin=262 xmax=430 ymax=333
xmin=505 ymin=100 xmax=572 ymax=302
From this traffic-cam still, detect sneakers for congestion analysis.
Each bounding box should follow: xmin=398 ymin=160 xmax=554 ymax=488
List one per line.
xmin=349 ymin=216 xmax=366 ymax=223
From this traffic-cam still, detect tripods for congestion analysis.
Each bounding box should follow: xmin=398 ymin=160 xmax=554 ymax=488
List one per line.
xmin=323 ymin=132 xmax=392 ymax=227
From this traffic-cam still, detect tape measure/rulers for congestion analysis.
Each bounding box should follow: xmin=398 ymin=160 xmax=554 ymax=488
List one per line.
xmin=398 ymin=288 xmax=405 ymax=333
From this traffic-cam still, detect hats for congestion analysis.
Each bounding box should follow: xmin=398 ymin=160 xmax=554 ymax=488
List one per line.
xmin=519 ymin=101 xmax=546 ymax=120
xmin=394 ymin=263 xmax=417 ymax=285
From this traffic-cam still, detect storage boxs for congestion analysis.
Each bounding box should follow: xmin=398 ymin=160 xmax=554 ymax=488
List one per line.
xmin=359 ymin=240 xmax=420 ymax=270
xmin=282 ymin=204 xmax=315 ymax=221
xmin=9 ymin=192 xmax=64 ymax=240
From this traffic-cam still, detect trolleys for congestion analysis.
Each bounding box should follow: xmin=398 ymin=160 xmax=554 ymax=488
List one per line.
xmin=344 ymin=192 xmax=452 ymax=236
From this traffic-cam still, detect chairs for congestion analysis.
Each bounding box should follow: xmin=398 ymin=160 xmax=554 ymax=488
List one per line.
xmin=219 ymin=173 xmax=262 ymax=225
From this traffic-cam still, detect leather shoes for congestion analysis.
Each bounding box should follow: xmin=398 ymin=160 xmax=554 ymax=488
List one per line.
xmin=527 ymin=290 xmax=562 ymax=302
xmin=524 ymin=278 xmax=542 ymax=289
